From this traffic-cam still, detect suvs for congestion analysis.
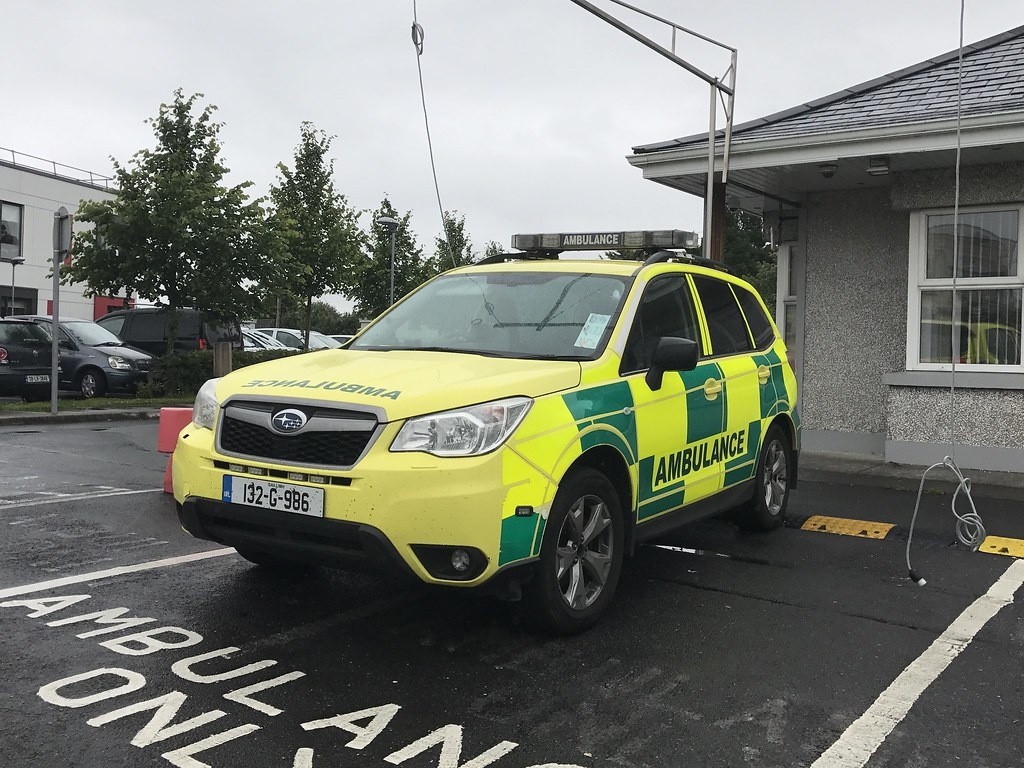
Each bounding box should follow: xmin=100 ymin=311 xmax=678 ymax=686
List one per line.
xmin=920 ymin=319 xmax=1022 ymax=365
xmin=90 ymin=307 xmax=245 ymax=356
xmin=172 ymin=230 xmax=805 ymax=635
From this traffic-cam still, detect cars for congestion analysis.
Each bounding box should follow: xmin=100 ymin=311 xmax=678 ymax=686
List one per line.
xmin=239 ymin=326 xmax=355 ymax=354
xmin=4 ymin=315 xmax=168 ymax=396
xmin=0 ymin=318 xmax=63 ymax=393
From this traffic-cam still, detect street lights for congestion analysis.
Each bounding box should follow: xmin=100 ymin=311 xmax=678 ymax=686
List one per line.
xmin=10 ymin=257 xmax=27 ymax=317
xmin=376 ymin=217 xmax=400 ymax=309
